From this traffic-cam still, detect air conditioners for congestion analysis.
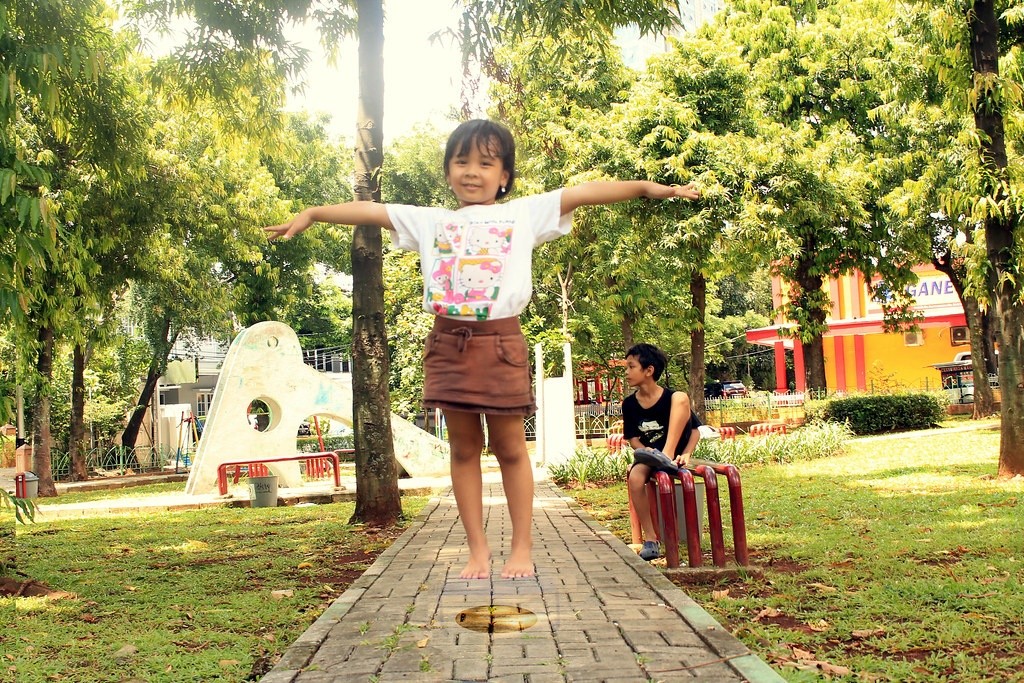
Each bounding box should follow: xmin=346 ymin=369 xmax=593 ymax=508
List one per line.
xmin=950 ymin=325 xmax=971 ymax=346
xmin=904 ymin=331 xmax=925 ymax=347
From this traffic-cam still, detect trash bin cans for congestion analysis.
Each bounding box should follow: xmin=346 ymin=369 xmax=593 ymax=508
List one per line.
xmin=696 ymin=425 xmax=720 ymax=455
xmin=646 ymin=482 xmax=705 ymax=541
xmin=16 ymin=472 xmax=39 ymax=499
xmin=247 ymin=475 xmax=279 ymax=507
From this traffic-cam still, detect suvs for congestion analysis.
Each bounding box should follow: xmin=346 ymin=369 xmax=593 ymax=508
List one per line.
xmin=704 ymin=380 xmax=749 ymax=399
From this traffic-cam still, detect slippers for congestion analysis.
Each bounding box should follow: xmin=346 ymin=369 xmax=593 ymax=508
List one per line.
xmin=633 ymin=448 xmax=678 ymax=475
xmin=639 ymin=540 xmax=659 ymax=560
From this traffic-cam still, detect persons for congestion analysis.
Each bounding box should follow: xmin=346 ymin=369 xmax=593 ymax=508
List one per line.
xmin=264 ymin=119 xmax=705 ymax=581
xmin=617 ymin=343 xmax=701 ymax=561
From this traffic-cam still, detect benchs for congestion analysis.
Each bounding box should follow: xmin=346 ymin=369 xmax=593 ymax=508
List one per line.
xmin=626 ymin=459 xmax=751 ymax=569
xmin=217 ymin=451 xmax=347 ymax=501
xmin=607 ymin=422 xmax=788 ymax=453
